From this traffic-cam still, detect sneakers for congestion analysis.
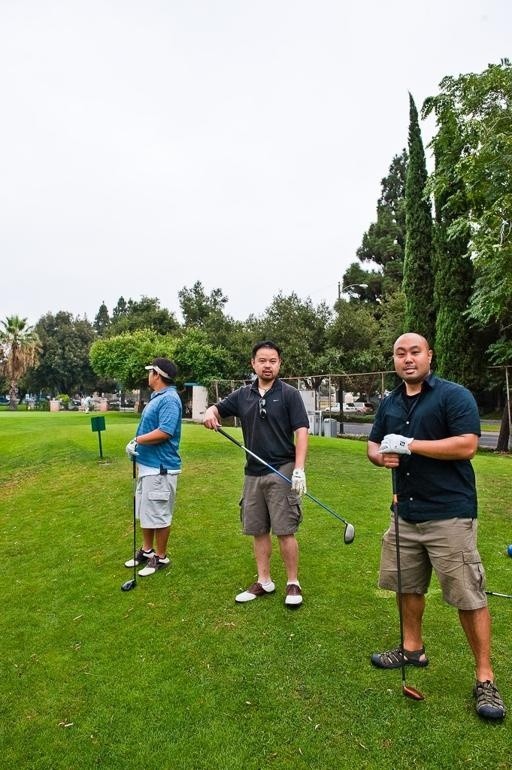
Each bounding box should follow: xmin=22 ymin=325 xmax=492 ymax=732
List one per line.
xmin=235 ymin=581 xmax=276 ymax=604
xmin=284 ymin=581 xmax=302 ymax=606
xmin=472 ymin=671 xmax=506 ymax=719
xmin=125 ymin=548 xmax=156 ymax=568
xmin=370 ymin=644 xmax=429 ymax=669
xmin=138 ymin=555 xmax=171 ymax=577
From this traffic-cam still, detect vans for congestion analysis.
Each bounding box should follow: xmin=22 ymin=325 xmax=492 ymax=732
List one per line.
xmin=352 ymin=402 xmax=373 ymax=413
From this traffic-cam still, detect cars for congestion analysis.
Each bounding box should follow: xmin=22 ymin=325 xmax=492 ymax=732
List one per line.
xmin=326 ymin=403 xmax=358 ymax=413
xmin=124 ymin=400 xmax=134 ymax=407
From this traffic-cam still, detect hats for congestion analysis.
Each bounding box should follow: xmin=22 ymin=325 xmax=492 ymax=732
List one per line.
xmin=144 ymin=358 xmax=178 ymax=381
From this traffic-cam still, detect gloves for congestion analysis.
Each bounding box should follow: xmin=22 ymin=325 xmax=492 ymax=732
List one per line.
xmin=377 ymin=433 xmax=415 ymax=456
xmin=126 ymin=437 xmax=140 ymax=458
xmin=290 ymin=469 xmax=307 ymax=497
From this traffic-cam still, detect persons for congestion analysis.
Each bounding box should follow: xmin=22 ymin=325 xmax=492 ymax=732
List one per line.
xmin=202 ymin=341 xmax=311 ymax=605
xmin=24 ymin=391 xmax=130 ymax=416
xmin=364 ymin=331 xmax=509 ymax=721
xmin=124 ymin=357 xmax=183 ymax=578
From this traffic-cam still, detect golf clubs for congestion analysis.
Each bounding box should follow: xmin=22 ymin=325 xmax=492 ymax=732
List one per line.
xmin=216 ymin=425 xmax=355 ymax=545
xmin=121 ymin=451 xmax=137 ymax=591
xmin=392 ymin=467 xmax=425 ymax=700
